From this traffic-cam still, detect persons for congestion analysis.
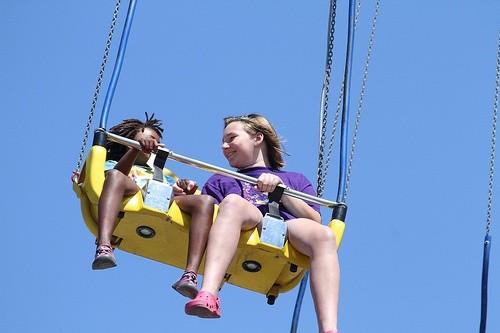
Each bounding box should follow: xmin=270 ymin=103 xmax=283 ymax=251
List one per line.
xmin=92 ymin=109 xmax=216 ymax=299
xmin=172 ymin=114 xmax=342 ymax=333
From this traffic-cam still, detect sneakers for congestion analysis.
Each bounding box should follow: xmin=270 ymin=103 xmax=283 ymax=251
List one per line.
xmin=91 ymin=244 xmax=117 ymax=270
xmin=171 ymin=270 xmax=198 ymax=299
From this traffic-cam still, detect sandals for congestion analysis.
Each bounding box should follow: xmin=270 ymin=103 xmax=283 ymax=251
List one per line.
xmin=185 ymin=291 xmax=222 ymax=319
